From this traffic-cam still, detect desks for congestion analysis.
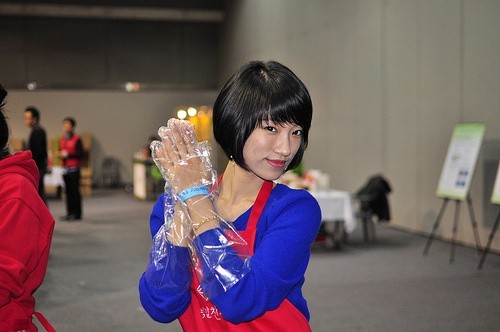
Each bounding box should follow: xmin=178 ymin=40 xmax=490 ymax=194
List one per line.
xmin=308 ymin=190 xmax=350 ymax=252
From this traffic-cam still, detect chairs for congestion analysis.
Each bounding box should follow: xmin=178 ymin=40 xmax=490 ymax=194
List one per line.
xmin=335 ymin=176 xmax=382 ymax=246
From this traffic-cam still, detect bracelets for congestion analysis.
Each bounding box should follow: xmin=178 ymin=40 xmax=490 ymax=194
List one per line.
xmin=192 ymin=213 xmax=222 ymax=230
xmin=176 ymin=184 xmax=213 ymax=204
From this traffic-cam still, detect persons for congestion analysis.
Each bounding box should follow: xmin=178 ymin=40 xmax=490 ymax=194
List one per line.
xmin=57 ymin=117 xmax=86 ymax=222
xmin=23 ymin=106 xmax=48 ymax=212
xmin=138 ymin=60 xmax=323 ymax=332
xmin=0 ymin=86 xmax=56 ymax=332
xmin=144 ymin=133 xmax=159 ymax=161
